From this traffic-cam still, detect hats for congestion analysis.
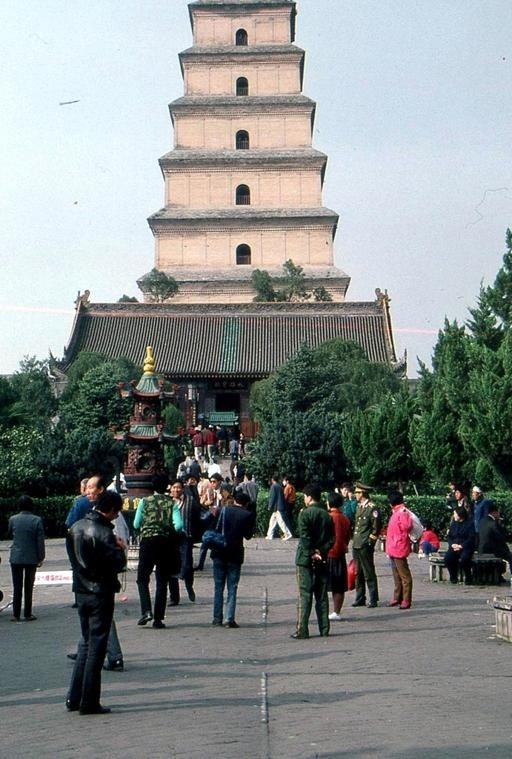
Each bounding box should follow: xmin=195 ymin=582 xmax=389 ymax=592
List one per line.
xmin=484 ymin=501 xmax=497 ymax=514
xmin=209 ymin=473 xmax=224 ymax=481
xmin=455 ymin=506 xmax=466 ymax=518
xmin=473 ymin=486 xmax=483 ymax=493
xmin=354 ymin=482 xmax=374 ymax=493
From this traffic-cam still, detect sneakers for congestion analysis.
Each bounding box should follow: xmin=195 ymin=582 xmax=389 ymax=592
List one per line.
xmin=103 ymin=659 xmax=124 ymax=670
xmin=292 ymin=631 xmax=304 ymax=638
xmin=329 ymin=612 xmax=342 ymax=621
xmin=168 ymin=588 xmax=195 ymax=607
xmin=266 ymin=535 xmax=272 ymax=539
xmin=452 ymin=579 xmax=471 ymax=585
xmin=138 ymin=611 xmax=165 ymax=628
xmin=66 ymin=699 xmax=111 ymax=715
xmin=282 ymin=533 xmax=292 ymax=539
xmin=352 ymin=601 xmax=411 ymax=609
xmin=68 ymin=653 xmax=78 ymax=658
xmin=192 ymin=566 xmax=203 ymax=571
xmin=418 ymin=552 xmax=432 ymax=558
xmin=10 ymin=615 xmax=37 ymax=621
xmin=213 ymin=620 xmax=239 ymax=629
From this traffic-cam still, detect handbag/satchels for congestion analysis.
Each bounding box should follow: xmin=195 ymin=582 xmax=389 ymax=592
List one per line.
xmin=314 ymin=558 xmax=341 ymax=584
xmin=471 ymin=560 xmax=505 ymax=586
xmin=407 ymin=509 xmax=423 ymax=542
xmin=203 ymin=529 xmax=228 ymax=550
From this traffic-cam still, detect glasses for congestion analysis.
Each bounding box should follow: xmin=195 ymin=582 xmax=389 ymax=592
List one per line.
xmin=210 ymin=481 xmax=217 ymax=483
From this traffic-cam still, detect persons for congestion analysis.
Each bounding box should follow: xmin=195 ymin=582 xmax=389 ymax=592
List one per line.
xmin=338 ymin=482 xmax=357 ymax=560
xmin=265 ymin=475 xmax=297 ymax=542
xmin=8 ymin=495 xmax=45 ymax=623
xmin=386 ymin=491 xmax=413 ymax=609
xmin=67 ymin=475 xmax=129 ymax=671
xmin=326 ymin=492 xmax=351 ymax=621
xmin=65 ymin=490 xmax=125 ymax=714
xmin=134 ymin=424 xmax=260 ymax=629
xmin=418 ymin=520 xmax=440 ymax=559
xmin=353 ymin=482 xmax=380 ymax=608
xmin=290 ymin=484 xmax=336 ymax=639
xmin=446 ymin=481 xmax=512 ymax=584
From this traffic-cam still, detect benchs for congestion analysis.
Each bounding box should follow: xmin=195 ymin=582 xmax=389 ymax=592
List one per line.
xmin=429 ymin=552 xmax=505 ymax=584
xmin=493 ymin=592 xmax=511 ymax=642
